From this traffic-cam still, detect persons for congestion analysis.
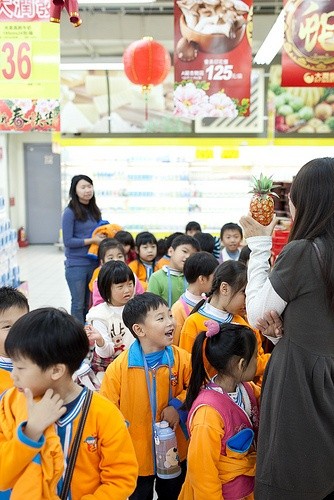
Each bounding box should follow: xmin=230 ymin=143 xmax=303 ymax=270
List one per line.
xmin=239 ymin=157 xmax=334 ymax=500
xmin=0 ymin=286 xmax=29 ymax=395
xmin=0 ymin=309 xmax=138 ymax=500
xmin=62 ymin=174 xmax=107 ymax=326
xmin=96 ymin=292 xmax=202 ymax=500
xmin=86 ymin=222 xmax=274 ymax=400
xmin=177 ymin=320 xmax=259 ymax=500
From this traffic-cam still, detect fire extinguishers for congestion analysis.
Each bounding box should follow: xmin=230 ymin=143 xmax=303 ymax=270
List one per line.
xmin=18 ymin=227 xmax=26 ymax=246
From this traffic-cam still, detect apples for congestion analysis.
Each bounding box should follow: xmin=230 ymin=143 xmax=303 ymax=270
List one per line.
xmin=275 ymin=92 xmax=313 ymax=120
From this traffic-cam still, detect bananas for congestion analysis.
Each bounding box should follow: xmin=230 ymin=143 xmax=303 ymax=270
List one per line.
xmin=288 ymin=86 xmax=324 ymax=107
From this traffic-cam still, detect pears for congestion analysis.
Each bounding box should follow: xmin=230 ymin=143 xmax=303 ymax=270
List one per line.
xmin=285 ymin=102 xmax=333 ymax=133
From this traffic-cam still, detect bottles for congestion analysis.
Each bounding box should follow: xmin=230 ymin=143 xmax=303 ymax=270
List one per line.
xmin=153 ymin=421 xmax=182 ymax=480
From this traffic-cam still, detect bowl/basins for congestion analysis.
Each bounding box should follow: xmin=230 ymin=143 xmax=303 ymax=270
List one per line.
xmin=179 ymin=13 xmax=246 ymax=55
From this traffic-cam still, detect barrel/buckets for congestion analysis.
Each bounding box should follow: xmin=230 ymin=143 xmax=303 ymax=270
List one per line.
xmin=272 ymin=230 xmax=289 ymax=259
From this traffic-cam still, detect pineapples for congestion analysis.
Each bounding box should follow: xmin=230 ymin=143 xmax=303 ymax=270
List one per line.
xmin=248 ymin=171 xmax=281 ymax=226
